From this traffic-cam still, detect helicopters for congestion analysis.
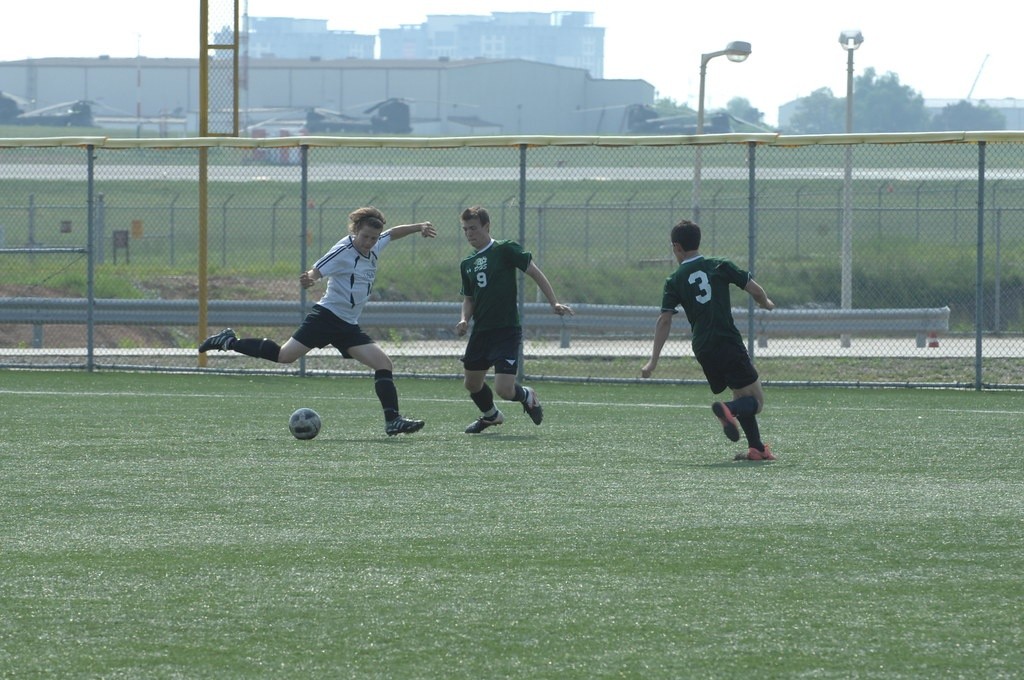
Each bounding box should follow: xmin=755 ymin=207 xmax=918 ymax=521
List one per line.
xmin=197 ymin=88 xmax=482 ymax=134
xmin=9 ymin=97 xmax=157 ymax=131
xmin=570 ymin=102 xmax=777 ymax=136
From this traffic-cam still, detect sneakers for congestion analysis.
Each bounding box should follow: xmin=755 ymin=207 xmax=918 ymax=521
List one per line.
xmin=748 ymin=444 xmax=777 ymax=460
xmin=199 ymin=328 xmax=236 ymax=353
xmin=520 ymin=386 xmax=544 ymax=425
xmin=465 ymin=410 xmax=504 ymax=433
xmin=712 ymin=401 xmax=740 ymax=442
xmin=385 ymin=416 xmax=425 ymax=437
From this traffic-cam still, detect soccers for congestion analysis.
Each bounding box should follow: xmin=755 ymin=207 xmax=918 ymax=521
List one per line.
xmin=289 ymin=408 xmax=320 ymax=440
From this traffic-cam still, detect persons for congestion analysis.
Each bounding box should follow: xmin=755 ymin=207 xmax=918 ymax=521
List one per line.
xmin=199 ymin=207 xmax=436 ymax=437
xmin=642 ymin=220 xmax=776 ymax=459
xmin=457 ymin=208 xmax=575 ymax=434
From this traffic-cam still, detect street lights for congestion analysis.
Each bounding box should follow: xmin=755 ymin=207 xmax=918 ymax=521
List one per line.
xmin=692 ymin=40 xmax=752 ymax=226
xmin=839 ymin=29 xmax=866 ymax=347
